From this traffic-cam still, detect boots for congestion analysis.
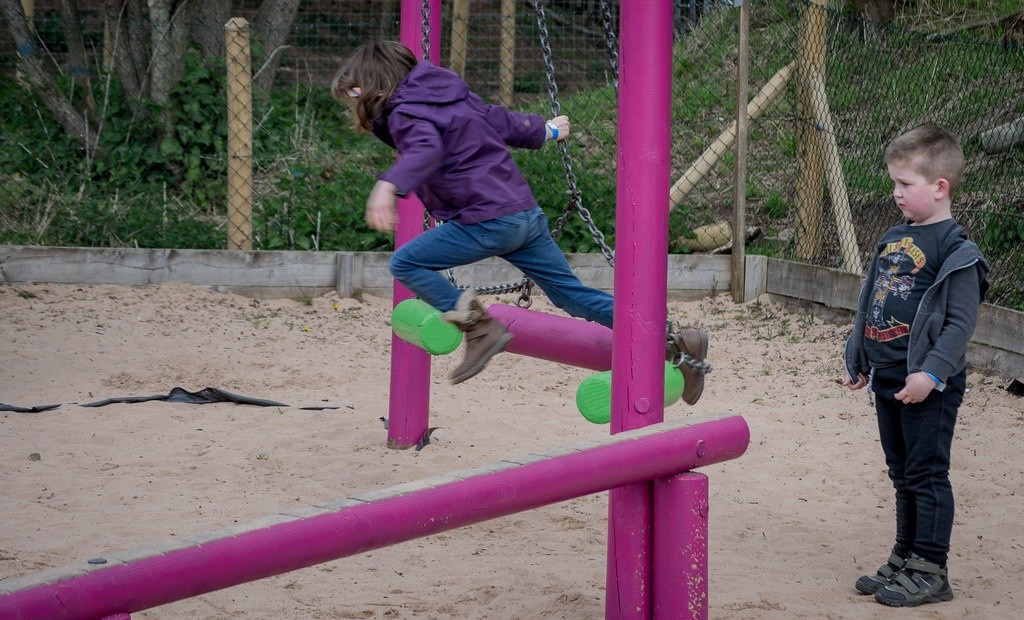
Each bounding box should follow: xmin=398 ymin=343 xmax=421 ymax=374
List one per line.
xmin=443 ymin=286 xmax=508 ymax=385
xmin=666 ymin=328 xmax=708 ymax=405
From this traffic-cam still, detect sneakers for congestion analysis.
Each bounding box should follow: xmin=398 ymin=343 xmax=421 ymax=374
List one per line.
xmin=875 ymin=555 xmax=954 ymax=608
xmin=855 ymin=542 xmax=909 ymax=595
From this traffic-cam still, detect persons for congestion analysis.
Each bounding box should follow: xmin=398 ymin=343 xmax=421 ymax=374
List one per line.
xmin=843 ymin=124 xmax=992 ymax=607
xmin=332 ymin=40 xmax=708 ymax=406
